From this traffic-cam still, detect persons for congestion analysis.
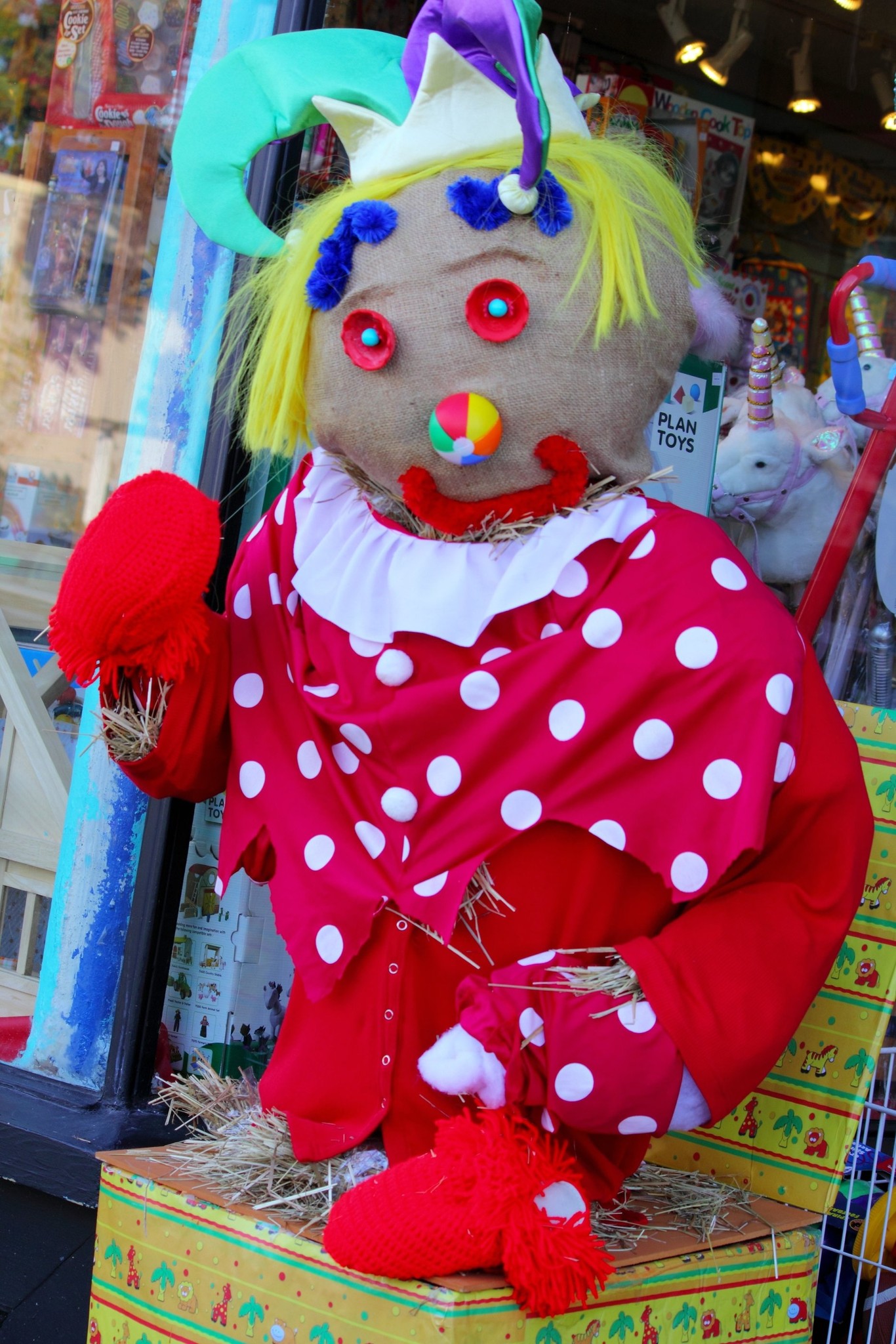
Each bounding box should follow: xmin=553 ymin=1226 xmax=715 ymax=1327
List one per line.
xmin=53 ymin=220 xmax=76 ymax=275
xmin=80 ymin=159 xmax=110 ymax=204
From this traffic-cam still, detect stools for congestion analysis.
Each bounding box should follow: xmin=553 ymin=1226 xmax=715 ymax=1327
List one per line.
xmin=86 ymin=696 xmax=896 ymax=1344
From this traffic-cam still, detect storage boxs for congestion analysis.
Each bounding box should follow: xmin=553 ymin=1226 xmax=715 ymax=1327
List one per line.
xmin=161 ymin=790 xmax=297 ymax=1085
xmin=575 ymin=73 xmax=755 ymax=259
xmin=0 ymin=0 xmax=204 ymax=573
xmin=16 ymin=641 xmax=84 ymax=766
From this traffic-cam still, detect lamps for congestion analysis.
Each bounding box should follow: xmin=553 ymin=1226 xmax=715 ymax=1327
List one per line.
xmin=868 ymin=71 xmax=896 ymax=131
xmin=785 ymin=13 xmax=822 ymax=113
xmin=654 ymin=0 xmax=708 ymax=64
xmin=697 ymin=0 xmax=754 ymax=87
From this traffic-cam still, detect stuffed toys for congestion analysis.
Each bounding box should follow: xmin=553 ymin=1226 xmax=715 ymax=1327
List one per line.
xmin=48 ymin=0 xmax=873 ymax=1319
xmin=710 ymin=352 xmax=896 ymax=619
